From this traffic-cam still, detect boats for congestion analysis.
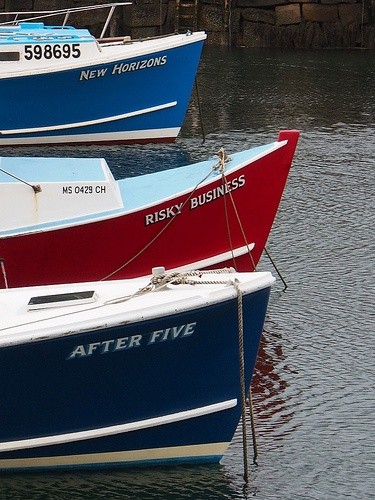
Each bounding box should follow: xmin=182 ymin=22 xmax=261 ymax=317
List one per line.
xmin=0 ymin=1 xmax=208 ymax=146
xmin=0 ymin=266 xmax=277 ymax=471
xmin=0 ymin=128 xmax=301 ymax=289
